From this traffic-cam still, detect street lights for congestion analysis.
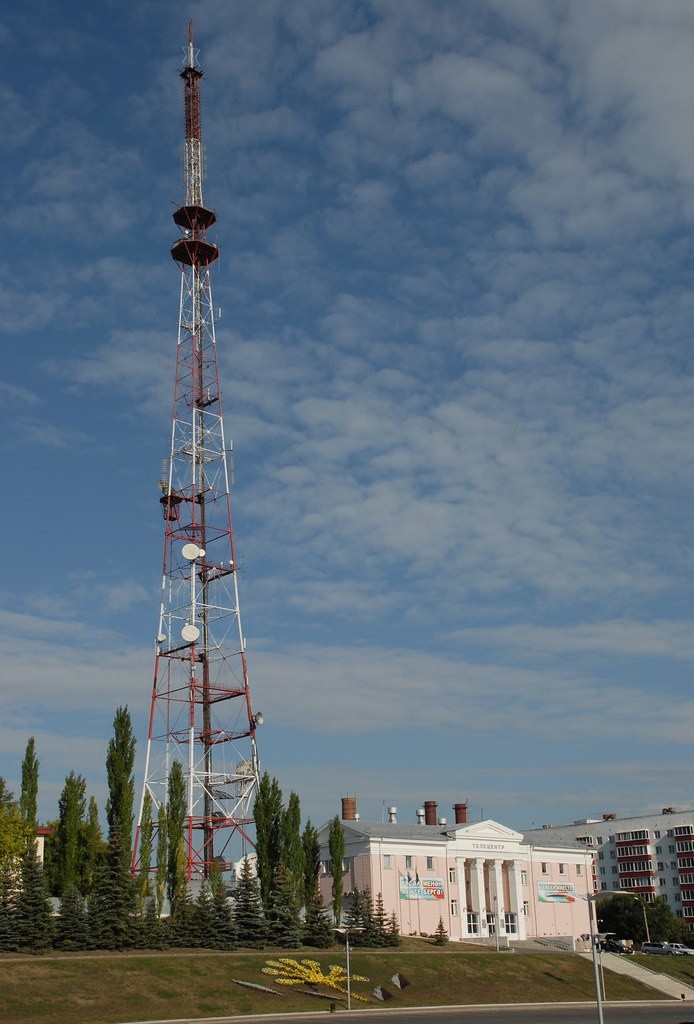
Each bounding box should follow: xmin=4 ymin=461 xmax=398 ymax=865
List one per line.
xmin=634 ymin=896 xmax=650 ymax=942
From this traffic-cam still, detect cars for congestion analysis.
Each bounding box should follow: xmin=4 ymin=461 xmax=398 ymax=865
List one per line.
xmin=641 ymin=941 xmax=678 ymax=956
xmin=667 ymin=943 xmax=694 ymax=957
xmin=596 ymin=940 xmax=633 ymax=954
